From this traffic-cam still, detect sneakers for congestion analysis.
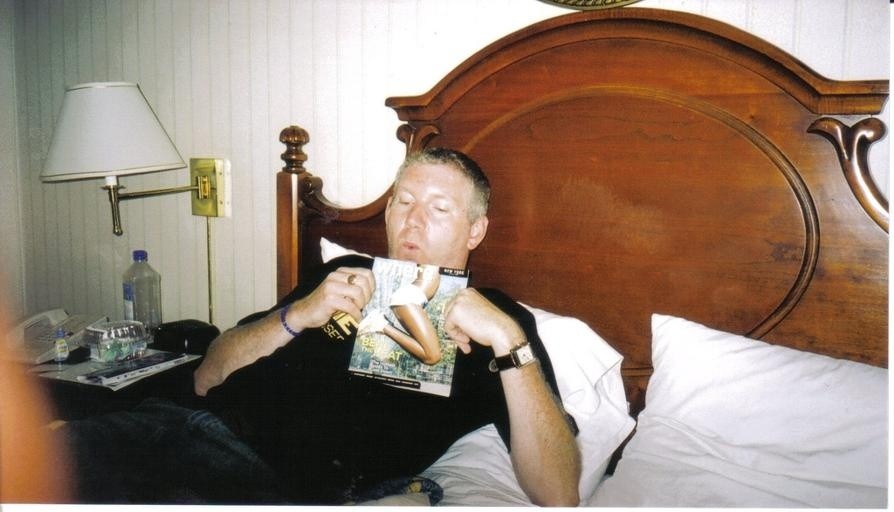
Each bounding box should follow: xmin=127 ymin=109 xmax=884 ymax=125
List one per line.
xmin=357 ymin=312 xmax=390 ymax=337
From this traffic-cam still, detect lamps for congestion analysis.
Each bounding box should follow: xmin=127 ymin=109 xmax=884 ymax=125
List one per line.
xmin=37 ymin=82 xmax=234 ymax=237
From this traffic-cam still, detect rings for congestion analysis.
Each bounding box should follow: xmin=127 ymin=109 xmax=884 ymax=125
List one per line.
xmin=346 ymin=273 xmax=357 ymax=284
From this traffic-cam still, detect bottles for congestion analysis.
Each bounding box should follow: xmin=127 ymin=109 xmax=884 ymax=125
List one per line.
xmin=121 ymin=250 xmax=162 ymax=344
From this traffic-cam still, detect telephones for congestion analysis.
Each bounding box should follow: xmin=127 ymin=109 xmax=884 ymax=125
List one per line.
xmin=0 ymin=308 xmax=109 ymax=365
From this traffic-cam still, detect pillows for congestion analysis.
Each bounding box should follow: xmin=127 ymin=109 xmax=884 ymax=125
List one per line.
xmin=319 ymin=237 xmax=374 ymax=264
xmin=591 ymin=314 xmax=890 ymax=506
xmin=418 ymin=300 xmax=637 ymax=507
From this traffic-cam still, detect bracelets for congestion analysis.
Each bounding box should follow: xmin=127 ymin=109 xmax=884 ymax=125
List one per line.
xmin=281 ymin=303 xmax=302 ymax=337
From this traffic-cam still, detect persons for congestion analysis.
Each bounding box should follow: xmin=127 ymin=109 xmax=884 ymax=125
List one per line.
xmin=56 ymin=149 xmax=582 ymax=505
xmin=357 ymin=264 xmax=442 ymax=365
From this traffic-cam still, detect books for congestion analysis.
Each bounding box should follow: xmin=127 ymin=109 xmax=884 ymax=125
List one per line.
xmin=348 ymin=254 xmax=471 ymax=397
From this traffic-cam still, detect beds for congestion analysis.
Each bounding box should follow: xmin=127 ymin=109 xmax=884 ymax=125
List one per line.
xmin=272 ymin=3 xmax=891 ymax=510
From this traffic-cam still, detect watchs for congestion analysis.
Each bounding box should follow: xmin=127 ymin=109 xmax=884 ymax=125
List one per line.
xmin=495 ymin=340 xmax=537 ymax=371
